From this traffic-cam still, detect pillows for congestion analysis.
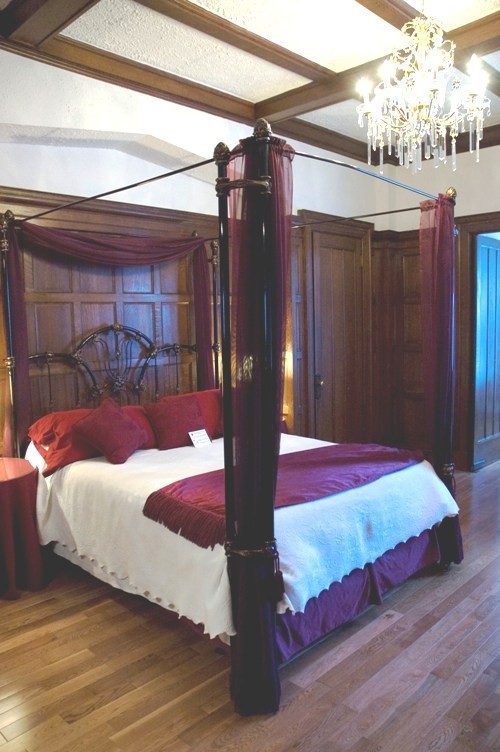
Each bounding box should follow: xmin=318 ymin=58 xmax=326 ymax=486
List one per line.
xmin=70 ymin=393 xmax=149 ymax=465
xmin=142 ymin=395 xmax=215 ymax=453
xmin=144 ymin=386 xmax=231 ymax=452
xmin=25 ymin=401 xmax=159 ymax=479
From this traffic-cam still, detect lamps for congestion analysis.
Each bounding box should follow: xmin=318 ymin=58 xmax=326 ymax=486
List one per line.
xmin=350 ymin=15 xmax=496 ymax=175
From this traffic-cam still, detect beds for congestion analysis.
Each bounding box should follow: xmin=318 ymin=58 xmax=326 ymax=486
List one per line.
xmin=14 ymin=321 xmax=467 ymax=672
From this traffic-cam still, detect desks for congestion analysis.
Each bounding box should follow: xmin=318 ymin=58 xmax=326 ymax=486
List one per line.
xmin=1 ymin=454 xmax=42 ymax=600
